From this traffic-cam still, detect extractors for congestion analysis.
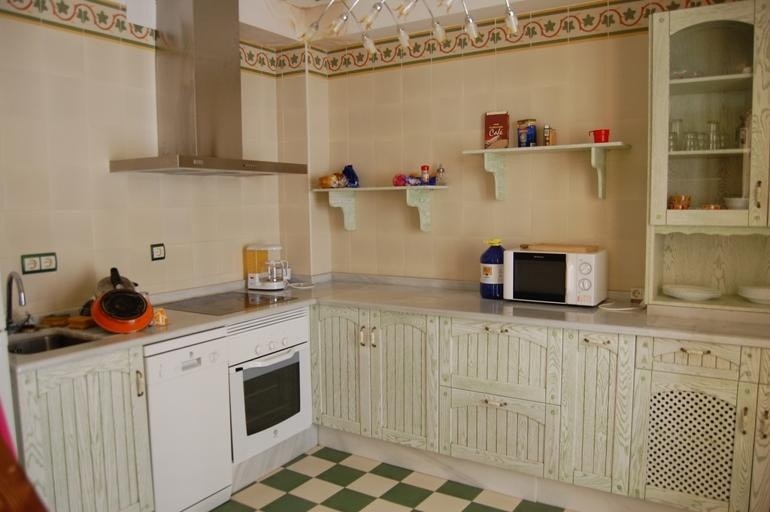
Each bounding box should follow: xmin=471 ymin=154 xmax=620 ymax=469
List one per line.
xmin=111 ymin=1 xmax=309 ymax=178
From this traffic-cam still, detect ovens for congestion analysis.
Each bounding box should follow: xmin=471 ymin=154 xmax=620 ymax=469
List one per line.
xmin=218 ymin=343 xmax=318 ymax=495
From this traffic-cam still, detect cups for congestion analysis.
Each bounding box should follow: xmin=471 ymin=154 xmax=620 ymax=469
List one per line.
xmin=671 ymin=113 xmax=748 ymax=150
xmin=588 ymin=129 xmax=609 ymax=144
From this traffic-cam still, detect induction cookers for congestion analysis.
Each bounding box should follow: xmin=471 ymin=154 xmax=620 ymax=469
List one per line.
xmin=159 ymin=291 xmax=312 ymax=366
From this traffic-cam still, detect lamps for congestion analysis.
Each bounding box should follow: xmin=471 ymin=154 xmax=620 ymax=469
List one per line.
xmin=299 ymin=1 xmax=521 ymax=56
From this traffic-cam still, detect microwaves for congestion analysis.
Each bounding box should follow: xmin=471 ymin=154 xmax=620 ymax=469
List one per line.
xmin=504 ymin=244 xmax=608 ymax=307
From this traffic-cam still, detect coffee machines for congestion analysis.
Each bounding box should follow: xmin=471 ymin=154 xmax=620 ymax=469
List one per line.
xmin=245 ymin=245 xmax=293 ymax=290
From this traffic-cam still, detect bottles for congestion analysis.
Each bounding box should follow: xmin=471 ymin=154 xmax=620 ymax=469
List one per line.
xmin=543 ymin=126 xmax=552 ymax=145
xmin=420 ymin=165 xmax=430 ymax=185
xmin=436 ymin=168 xmax=445 ymax=186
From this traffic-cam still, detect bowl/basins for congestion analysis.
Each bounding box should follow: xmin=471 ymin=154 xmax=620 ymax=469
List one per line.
xmin=666 ymin=193 xmax=746 ymax=210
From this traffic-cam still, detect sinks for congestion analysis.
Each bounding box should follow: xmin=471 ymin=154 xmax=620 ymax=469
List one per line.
xmin=6 ymin=330 xmax=102 ymax=355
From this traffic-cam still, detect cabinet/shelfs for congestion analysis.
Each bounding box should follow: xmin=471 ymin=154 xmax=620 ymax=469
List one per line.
xmin=630 ymin=330 xmax=770 ymax=511
xmin=641 ymin=0 xmax=770 ymax=325
xmin=559 ymin=323 xmax=636 ymax=498
xmin=438 ymin=313 xmax=561 ymax=481
xmin=8 ymin=340 xmax=154 ymax=511
xmin=307 ymin=299 xmax=437 ymax=455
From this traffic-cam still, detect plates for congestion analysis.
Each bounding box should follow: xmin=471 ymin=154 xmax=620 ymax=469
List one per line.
xmin=291 ymin=282 xmax=316 ymax=289
xmin=662 ymin=283 xmax=770 ymax=306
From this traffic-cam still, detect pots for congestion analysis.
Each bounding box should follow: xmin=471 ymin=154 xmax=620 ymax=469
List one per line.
xmin=79 ymin=267 xmax=154 ymax=333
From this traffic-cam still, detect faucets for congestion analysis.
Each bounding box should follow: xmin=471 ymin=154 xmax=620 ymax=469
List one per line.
xmin=6 ymin=271 xmax=27 ymax=333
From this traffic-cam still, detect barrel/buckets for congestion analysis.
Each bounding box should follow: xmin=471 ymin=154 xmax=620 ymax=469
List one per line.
xmin=480 ymin=238 xmax=506 ymax=299
